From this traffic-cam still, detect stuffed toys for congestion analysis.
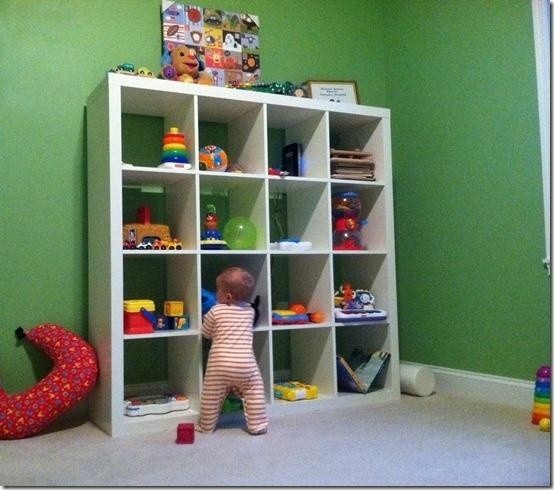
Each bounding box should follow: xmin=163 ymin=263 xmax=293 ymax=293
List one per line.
xmin=164 ymin=43 xmax=214 ymax=86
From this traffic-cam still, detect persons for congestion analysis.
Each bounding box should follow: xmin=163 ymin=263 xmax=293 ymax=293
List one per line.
xmin=194 ymin=266 xmax=269 ymax=435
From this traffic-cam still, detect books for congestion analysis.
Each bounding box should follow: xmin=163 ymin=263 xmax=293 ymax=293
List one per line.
xmin=339 ymin=347 xmax=392 ymax=394
xmin=280 ymin=143 xmax=302 ymax=179
xmin=329 ymin=144 xmax=375 ymax=182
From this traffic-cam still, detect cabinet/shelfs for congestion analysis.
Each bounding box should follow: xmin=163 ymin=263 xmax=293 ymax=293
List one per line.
xmin=87 ymin=73 xmax=401 ymax=438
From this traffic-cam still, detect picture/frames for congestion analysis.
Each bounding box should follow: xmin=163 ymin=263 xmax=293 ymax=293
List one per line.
xmin=306 ymin=79 xmax=358 ymax=105
